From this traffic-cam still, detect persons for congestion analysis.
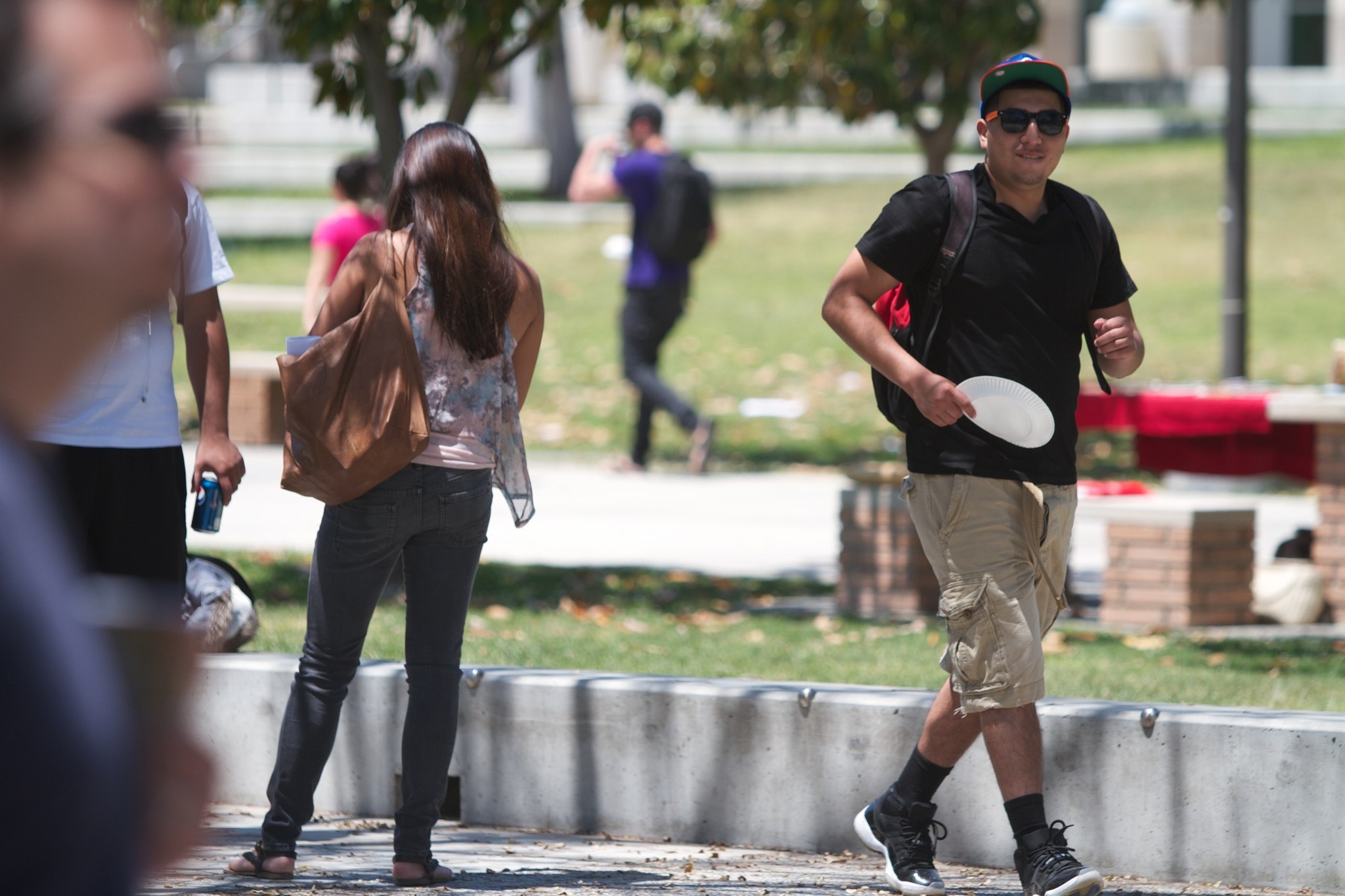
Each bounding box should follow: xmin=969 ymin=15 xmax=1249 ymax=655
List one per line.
xmin=821 ymin=54 xmax=1144 ymax=896
xmin=567 ymin=103 xmax=714 ymax=474
xmin=0 ymin=0 xmax=545 ymax=895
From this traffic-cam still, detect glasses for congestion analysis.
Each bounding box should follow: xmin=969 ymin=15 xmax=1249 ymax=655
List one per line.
xmin=985 ymin=108 xmax=1068 ymax=136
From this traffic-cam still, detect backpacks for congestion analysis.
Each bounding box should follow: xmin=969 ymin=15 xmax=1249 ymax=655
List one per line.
xmin=870 ymin=170 xmax=1111 ymax=434
xmin=642 ymin=155 xmax=711 ymax=268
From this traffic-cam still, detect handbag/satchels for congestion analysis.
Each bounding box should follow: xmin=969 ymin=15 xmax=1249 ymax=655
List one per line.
xmin=276 ymin=229 xmax=430 ymax=506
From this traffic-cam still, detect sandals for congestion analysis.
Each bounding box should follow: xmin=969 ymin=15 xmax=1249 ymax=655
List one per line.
xmin=392 ymin=854 xmax=457 ymax=886
xmin=223 ymin=840 xmax=297 ymax=880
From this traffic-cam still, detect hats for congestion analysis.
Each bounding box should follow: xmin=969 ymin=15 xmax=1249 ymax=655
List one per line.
xmin=980 ymin=53 xmax=1071 ymax=122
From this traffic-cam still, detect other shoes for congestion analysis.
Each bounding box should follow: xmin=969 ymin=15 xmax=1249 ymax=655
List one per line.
xmin=607 ymin=457 xmax=647 ymax=472
xmin=691 ymin=420 xmax=717 ymax=471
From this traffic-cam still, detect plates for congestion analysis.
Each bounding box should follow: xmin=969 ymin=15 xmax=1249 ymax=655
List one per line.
xmin=954 ymin=375 xmax=1055 ymax=448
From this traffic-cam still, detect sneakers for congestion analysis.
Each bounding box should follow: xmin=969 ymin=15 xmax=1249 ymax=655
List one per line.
xmin=853 ymin=781 xmax=948 ymax=896
xmin=1014 ymin=820 xmax=1104 ymax=896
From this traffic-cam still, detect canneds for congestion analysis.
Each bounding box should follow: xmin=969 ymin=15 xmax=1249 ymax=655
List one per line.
xmin=191 ymin=478 xmax=224 ymax=534
